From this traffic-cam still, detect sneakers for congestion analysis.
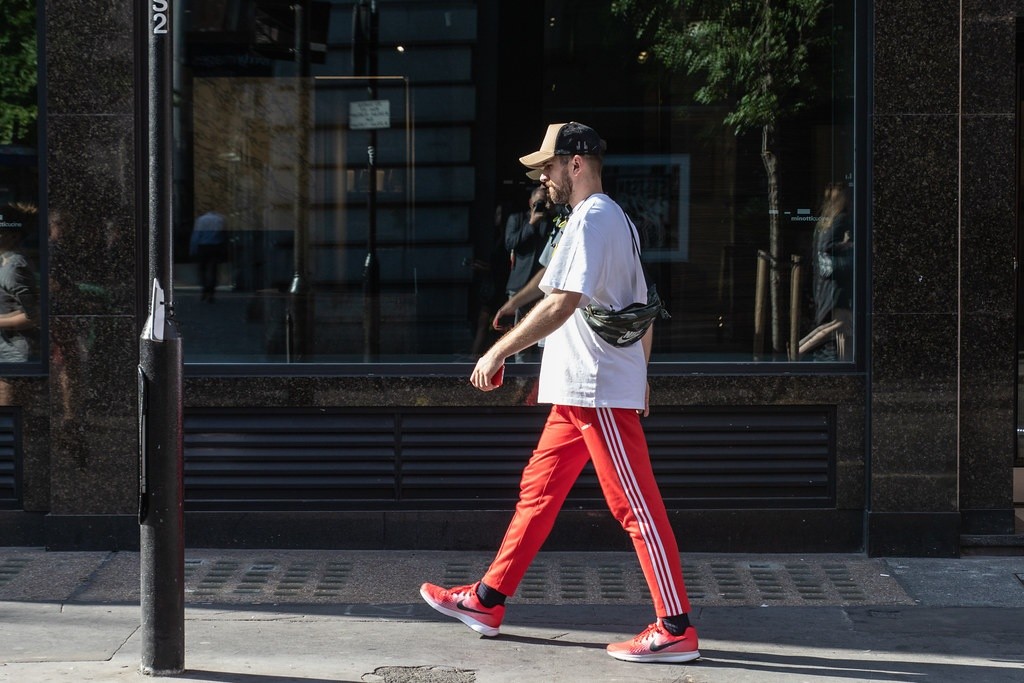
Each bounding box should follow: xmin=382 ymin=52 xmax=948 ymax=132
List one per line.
xmin=420 ymin=581 xmax=506 ymax=636
xmin=606 ymin=618 xmax=701 ymax=662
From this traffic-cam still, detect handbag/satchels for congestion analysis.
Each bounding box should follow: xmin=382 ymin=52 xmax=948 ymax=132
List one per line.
xmin=579 ymin=193 xmax=672 ymax=348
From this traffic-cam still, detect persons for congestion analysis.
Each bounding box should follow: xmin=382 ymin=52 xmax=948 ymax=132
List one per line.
xmin=790 ymin=180 xmax=857 ymax=366
xmin=0 ymin=206 xmax=129 ymax=432
xmin=491 ymin=166 xmax=574 ymax=407
xmin=503 ymin=187 xmax=560 ymax=406
xmin=189 ymin=198 xmax=230 ymax=309
xmin=418 ymin=121 xmax=701 ymax=664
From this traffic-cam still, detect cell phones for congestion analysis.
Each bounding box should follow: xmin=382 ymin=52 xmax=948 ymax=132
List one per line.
xmin=496 ymin=315 xmax=515 ymax=328
xmin=490 ymin=365 xmax=505 ymax=386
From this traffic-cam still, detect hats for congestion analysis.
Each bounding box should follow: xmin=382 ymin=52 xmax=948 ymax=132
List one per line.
xmin=519 ymin=122 xmax=606 ymax=169
xmin=526 ymin=170 xmax=544 ymax=180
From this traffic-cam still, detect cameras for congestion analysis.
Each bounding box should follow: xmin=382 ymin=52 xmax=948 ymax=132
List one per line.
xmin=532 ymin=199 xmax=547 ymax=213
